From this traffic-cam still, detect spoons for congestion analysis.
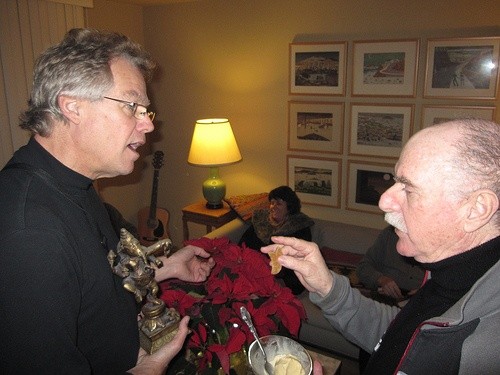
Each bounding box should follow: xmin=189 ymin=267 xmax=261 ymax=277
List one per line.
xmin=240 ymin=306 xmax=277 ymax=375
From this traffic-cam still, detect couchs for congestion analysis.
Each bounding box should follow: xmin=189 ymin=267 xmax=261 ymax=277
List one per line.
xmin=201 ymin=217 xmax=383 ymax=359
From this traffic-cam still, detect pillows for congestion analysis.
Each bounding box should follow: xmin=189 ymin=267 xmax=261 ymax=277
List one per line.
xmin=226 ymin=193 xmax=270 ymax=222
xmin=327 ymin=261 xmax=363 ymax=288
xmin=321 ymin=246 xmax=363 ymax=264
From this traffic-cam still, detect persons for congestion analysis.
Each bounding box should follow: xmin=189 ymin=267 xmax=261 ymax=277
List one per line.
xmin=0 ymin=27 xmax=216 ymax=375
xmin=260 ymin=119 xmax=500 ymax=375
xmin=357 ymin=225 xmax=432 ymax=308
xmin=239 ymin=186 xmax=315 ymax=296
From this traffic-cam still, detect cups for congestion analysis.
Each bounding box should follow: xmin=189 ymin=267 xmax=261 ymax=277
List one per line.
xmin=248 ymin=335 xmax=313 ymax=375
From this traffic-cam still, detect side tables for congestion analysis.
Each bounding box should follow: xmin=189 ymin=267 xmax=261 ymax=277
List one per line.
xmin=180 ymin=200 xmax=238 ymax=248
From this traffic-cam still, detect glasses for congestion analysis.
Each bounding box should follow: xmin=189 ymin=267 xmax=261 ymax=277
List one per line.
xmin=104 ymin=95 xmax=156 ymax=123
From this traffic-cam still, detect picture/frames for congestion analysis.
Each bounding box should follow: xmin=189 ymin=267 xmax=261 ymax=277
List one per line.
xmin=348 ymin=102 xmax=415 ymax=159
xmin=288 ymin=100 xmax=345 ymax=154
xmin=346 ymin=159 xmax=397 ymax=215
xmin=286 ymin=155 xmax=343 ymax=208
xmin=288 ymin=41 xmax=348 ymax=97
xmin=420 ymin=104 xmax=497 ymax=131
xmin=350 ymin=39 xmax=420 ymax=98
xmin=423 ymin=36 xmax=500 ymax=100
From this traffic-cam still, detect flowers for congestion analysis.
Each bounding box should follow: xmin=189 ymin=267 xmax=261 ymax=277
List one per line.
xmin=159 ymin=237 xmax=308 ymax=375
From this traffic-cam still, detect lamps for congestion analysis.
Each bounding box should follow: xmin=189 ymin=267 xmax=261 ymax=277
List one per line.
xmin=187 ymin=117 xmax=243 ymax=209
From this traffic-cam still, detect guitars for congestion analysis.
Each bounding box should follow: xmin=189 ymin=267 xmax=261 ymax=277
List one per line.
xmin=137 ymin=150 xmax=172 ymax=256
